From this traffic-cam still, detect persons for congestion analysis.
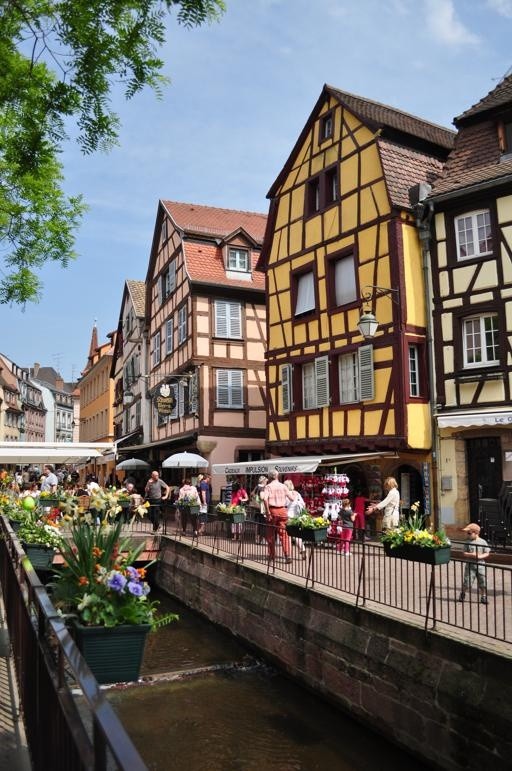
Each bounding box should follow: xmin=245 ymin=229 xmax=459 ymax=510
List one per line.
xmin=337 ymin=498 xmax=357 ymax=556
xmin=353 ymin=491 xmax=381 ymax=541
xmin=459 ymin=523 xmax=491 ymax=604
xmin=365 ymin=477 xmax=400 ymax=535
xmin=9 ymin=465 xmax=307 ymax=564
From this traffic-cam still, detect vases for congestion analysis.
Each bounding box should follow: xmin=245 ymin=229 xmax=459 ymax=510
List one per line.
xmin=21 ymin=540 xmax=54 ymax=571
xmin=179 ymin=505 xmax=199 ymax=515
xmin=217 ymin=511 xmax=245 ymax=524
xmin=286 ymin=526 xmax=327 ymax=542
xmin=118 ymin=501 xmax=130 ymax=508
xmin=71 ymin=617 xmax=152 ymax=684
xmin=39 ymin=499 xmax=59 ymax=508
xmin=8 ymin=520 xmax=24 ymax=533
xmin=383 ymin=541 xmax=451 ymax=565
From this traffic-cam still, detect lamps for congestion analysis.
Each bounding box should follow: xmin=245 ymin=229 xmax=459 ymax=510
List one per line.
xmin=123 ymin=375 xmax=149 ymax=404
xmin=357 ymin=285 xmax=400 ymax=342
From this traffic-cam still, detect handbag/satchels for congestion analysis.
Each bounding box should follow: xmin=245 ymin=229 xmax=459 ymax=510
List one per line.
xmin=382 ymin=516 xmax=398 ymax=530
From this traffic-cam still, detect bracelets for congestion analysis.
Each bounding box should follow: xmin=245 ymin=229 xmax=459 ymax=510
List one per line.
xmin=373 ymin=507 xmax=375 ymax=512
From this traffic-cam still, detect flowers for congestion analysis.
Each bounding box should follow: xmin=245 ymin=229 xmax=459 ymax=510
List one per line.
xmin=285 ymin=507 xmax=331 ymax=529
xmin=39 ymin=490 xmax=59 ymax=499
xmin=47 ymin=485 xmax=181 ymax=633
xmin=172 ymin=493 xmax=200 ymax=507
xmin=116 ymin=495 xmax=130 ymax=501
xmin=381 ymin=501 xmax=451 ymax=550
xmin=16 ymin=497 xmax=63 ymax=548
xmin=216 ymin=502 xmax=246 ymax=514
xmin=7 ymin=500 xmax=26 ymax=522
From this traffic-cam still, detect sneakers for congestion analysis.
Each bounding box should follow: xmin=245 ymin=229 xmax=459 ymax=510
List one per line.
xmin=459 ymin=592 xmax=490 ymax=605
xmin=231 ymin=537 xmax=354 ymax=564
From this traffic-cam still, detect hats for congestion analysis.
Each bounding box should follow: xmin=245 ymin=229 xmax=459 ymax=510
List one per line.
xmin=462 ymin=524 xmax=480 ymax=532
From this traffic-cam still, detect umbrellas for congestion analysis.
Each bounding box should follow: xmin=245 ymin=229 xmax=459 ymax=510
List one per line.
xmin=116 ymin=457 xmax=152 ymax=471
xmin=162 ymin=450 xmax=209 ymax=482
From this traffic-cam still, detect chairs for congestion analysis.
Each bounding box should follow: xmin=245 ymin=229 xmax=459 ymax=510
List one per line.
xmin=479 ymin=482 xmax=512 ymax=549
